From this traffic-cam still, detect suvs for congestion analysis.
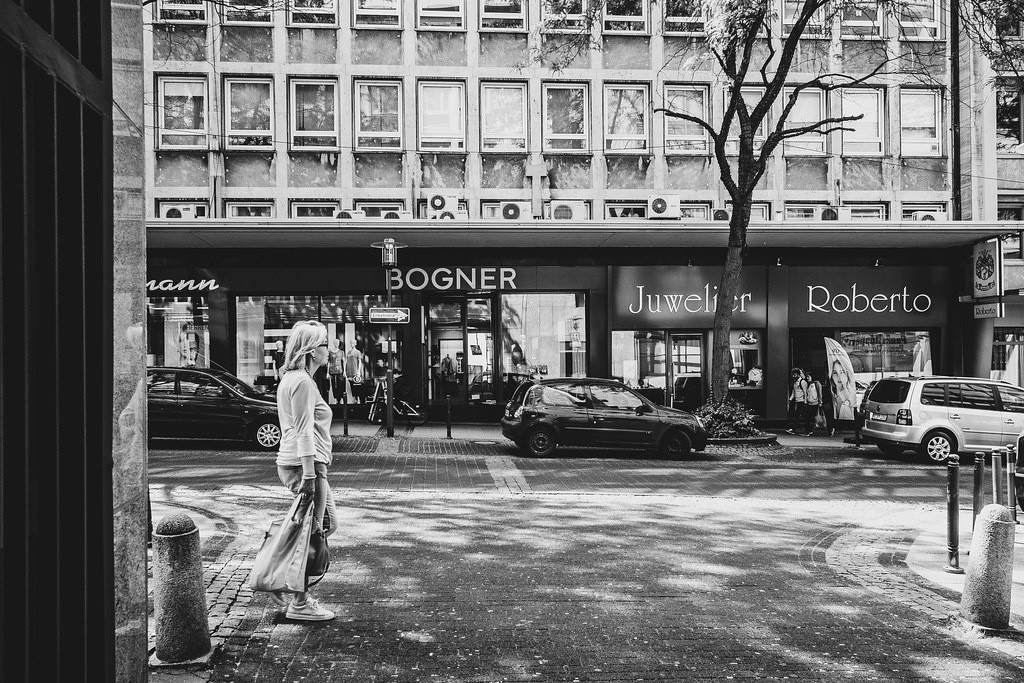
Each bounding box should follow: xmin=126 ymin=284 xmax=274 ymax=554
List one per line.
xmin=500 ymin=377 xmax=710 ymax=458
xmin=467 ymin=370 xmax=537 ymax=404
xmin=859 ymin=374 xmax=1024 ymax=464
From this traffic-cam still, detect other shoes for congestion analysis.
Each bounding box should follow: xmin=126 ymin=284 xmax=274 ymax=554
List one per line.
xmin=786 ymin=429 xmax=796 ymax=436
xmin=799 ymin=432 xmax=813 ymax=437
xmin=831 ymin=427 xmax=835 ymax=436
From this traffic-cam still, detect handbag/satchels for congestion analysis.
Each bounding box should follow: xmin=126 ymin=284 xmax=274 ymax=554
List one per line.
xmin=305 ymin=497 xmax=330 ymax=587
xmin=249 ymin=493 xmax=314 ymax=594
xmin=815 ymin=405 xmax=827 ymax=428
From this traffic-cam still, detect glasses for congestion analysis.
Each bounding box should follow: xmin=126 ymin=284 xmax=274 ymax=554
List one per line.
xmin=318 ymin=342 xmax=328 ymax=349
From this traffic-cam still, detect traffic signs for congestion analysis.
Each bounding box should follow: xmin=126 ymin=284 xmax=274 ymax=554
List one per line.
xmin=369 ymin=308 xmax=411 ymax=324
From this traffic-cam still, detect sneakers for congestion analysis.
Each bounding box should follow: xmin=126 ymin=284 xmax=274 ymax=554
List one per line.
xmin=286 ymin=597 xmax=334 ymax=621
xmin=265 ymin=590 xmax=287 ymax=607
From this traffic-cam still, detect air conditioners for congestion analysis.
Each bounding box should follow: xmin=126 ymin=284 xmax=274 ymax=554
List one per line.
xmin=162 ymin=205 xmax=195 ymax=218
xmin=813 ymin=206 xmax=851 ymax=221
xmin=913 ymin=211 xmax=948 ymax=222
xmin=427 ymin=193 xmax=458 ymax=215
xmin=500 ymin=201 xmax=531 ymax=220
xmin=333 ymin=210 xmax=366 ymax=218
xmin=381 ymin=211 xmax=413 ymax=220
xmin=649 ymin=195 xmax=681 ymax=219
xmin=551 ymin=201 xmax=585 ymax=221
xmin=427 ymin=212 xmax=437 ymax=220
xmin=436 ymin=210 xmax=468 ymax=220
xmin=711 ymin=208 xmax=733 ymax=220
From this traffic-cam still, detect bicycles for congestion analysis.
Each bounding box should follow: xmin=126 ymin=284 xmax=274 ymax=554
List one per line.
xmin=367 ymin=376 xmax=428 ymax=426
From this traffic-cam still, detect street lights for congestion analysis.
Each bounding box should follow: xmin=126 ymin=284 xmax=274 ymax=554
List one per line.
xmin=370 ymin=237 xmax=409 ymax=434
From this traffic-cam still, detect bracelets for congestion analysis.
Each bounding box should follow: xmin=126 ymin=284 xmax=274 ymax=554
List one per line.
xmin=302 ymin=473 xmax=317 ymax=479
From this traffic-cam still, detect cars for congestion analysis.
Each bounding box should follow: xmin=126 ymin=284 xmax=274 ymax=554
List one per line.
xmin=147 ymin=367 xmax=281 ymax=450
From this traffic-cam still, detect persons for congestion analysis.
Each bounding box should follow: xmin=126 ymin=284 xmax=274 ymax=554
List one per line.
xmin=786 ymin=369 xmax=836 ymax=437
xmin=266 ymin=320 xmax=339 ymax=622
xmin=326 ymin=339 xmax=362 ymax=404
xmin=178 ymin=324 xmax=191 ymax=366
xmin=829 ymin=357 xmax=856 ymax=420
xmin=271 ymin=341 xmax=286 ymax=383
